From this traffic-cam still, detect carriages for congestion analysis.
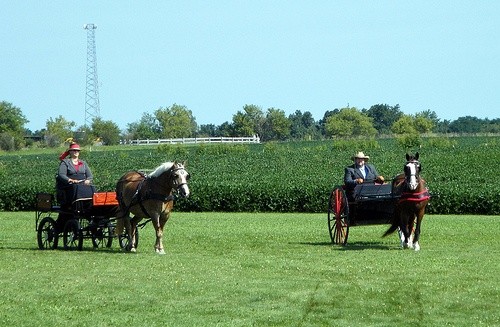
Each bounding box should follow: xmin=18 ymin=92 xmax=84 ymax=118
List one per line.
xmin=36 ymin=158 xmax=190 ymax=256
xmin=328 ymin=153 xmax=429 ymax=250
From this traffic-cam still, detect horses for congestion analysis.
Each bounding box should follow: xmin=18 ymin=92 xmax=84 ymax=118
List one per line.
xmin=380 ymin=152 xmax=428 ymax=251
xmin=113 ymin=159 xmax=190 ymax=255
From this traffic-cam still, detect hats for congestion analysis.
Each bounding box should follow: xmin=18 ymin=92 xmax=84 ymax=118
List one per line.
xmin=351 ymin=151 xmax=369 ymax=161
xmin=59 ymin=144 xmax=82 ymax=160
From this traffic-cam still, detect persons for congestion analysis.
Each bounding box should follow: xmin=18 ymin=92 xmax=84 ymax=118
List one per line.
xmin=56 ymin=141 xmax=96 ymax=215
xmin=343 ymin=151 xmax=385 ymax=200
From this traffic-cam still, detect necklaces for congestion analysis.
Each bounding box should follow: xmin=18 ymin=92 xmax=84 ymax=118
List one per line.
xmin=73 ymin=160 xmax=78 ymax=164
